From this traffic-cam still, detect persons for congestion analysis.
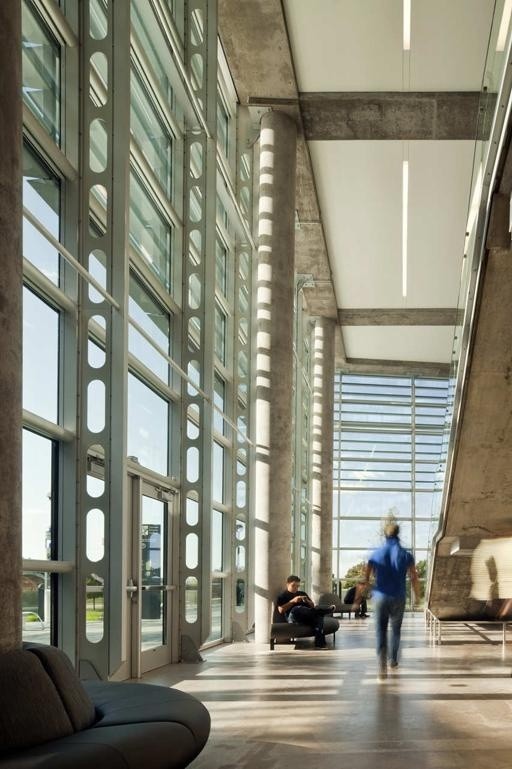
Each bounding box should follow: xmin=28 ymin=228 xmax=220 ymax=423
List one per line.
xmin=344 ymin=583 xmax=365 ymax=619
xmin=359 ymin=582 xmax=370 ymax=618
xmin=359 ymin=522 xmax=422 ymax=674
xmin=276 ymin=576 xmax=336 ymax=650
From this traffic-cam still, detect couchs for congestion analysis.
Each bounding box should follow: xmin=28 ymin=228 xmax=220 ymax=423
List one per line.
xmin=315 ymin=592 xmax=362 ymax=618
xmin=2 ymin=637 xmax=214 ymax=769
xmin=269 ymin=604 xmax=343 ymax=649
